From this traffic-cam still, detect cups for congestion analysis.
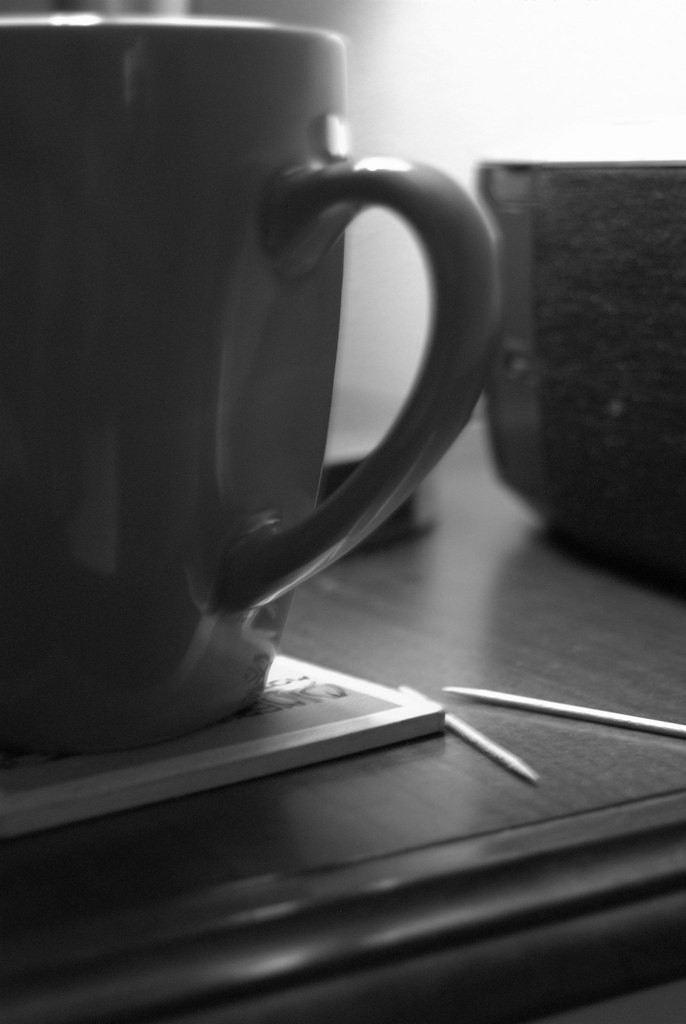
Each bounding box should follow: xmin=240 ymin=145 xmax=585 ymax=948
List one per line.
xmin=0 ymin=14 xmax=503 ymax=760
